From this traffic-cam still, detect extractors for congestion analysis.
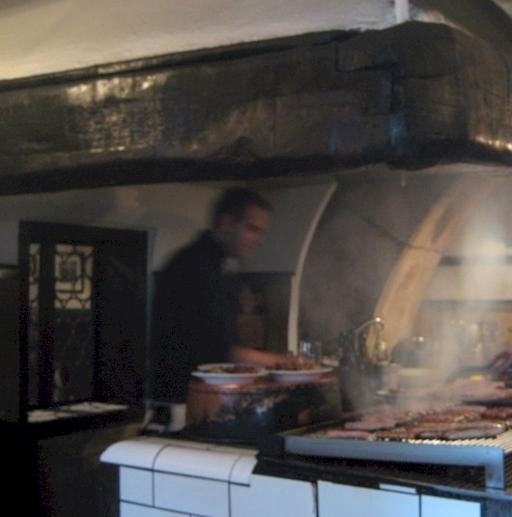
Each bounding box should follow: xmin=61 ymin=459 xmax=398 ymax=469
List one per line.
xmin=0 ymin=1 xmax=511 ymax=195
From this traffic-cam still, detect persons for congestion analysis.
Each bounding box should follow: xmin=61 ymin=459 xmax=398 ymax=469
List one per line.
xmin=147 ymin=186 xmax=315 ymax=404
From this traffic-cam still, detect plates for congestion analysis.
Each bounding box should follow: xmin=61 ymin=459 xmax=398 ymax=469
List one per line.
xmin=193 ymin=361 xmax=330 ymax=386
